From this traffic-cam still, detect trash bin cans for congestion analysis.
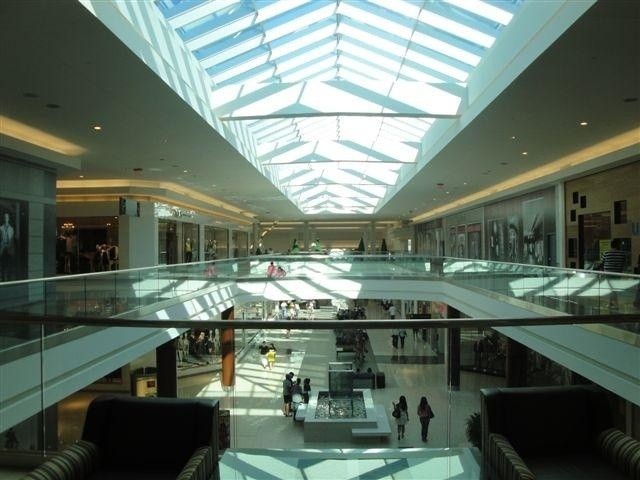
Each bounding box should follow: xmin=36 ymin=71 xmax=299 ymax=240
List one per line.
xmin=220 ymin=410 xmax=231 ymax=450
xmin=377 ymin=371 xmax=386 ymax=388
xmin=130 ymin=366 xmax=157 ymax=397
xmin=392 ymin=335 xmax=400 ymax=348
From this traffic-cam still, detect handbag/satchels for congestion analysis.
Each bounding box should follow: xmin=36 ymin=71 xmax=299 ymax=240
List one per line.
xmin=427 ymin=404 xmax=434 ymax=418
xmin=392 ymin=408 xmax=401 ymax=417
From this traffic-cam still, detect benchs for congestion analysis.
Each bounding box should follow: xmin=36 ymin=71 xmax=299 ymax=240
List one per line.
xmin=351 ymin=403 xmax=391 ymax=438
xmin=294 ymin=402 xmax=308 ymax=421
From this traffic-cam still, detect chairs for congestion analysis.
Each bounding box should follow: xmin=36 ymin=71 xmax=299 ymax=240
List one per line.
xmin=477 ymin=381 xmax=639 ymax=480
xmin=16 ymin=393 xmax=224 ymax=480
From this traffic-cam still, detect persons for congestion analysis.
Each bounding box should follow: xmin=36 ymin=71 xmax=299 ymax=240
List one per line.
xmin=184 ymin=238 xmax=192 ymax=263
xmin=98 ymin=243 xmax=111 ymax=271
xmin=593 ymin=238 xmax=628 ymax=312
xmin=0 ymin=210 xmax=16 ymax=281
xmin=178 ymin=329 xmax=222 ymax=366
xmin=205 ymin=238 xmax=216 ymax=262
xmin=473 ymin=339 xmax=483 ymax=370
xmin=391 ymin=396 xmax=411 ymax=441
xmin=417 ymin=396 xmax=434 ymax=443
xmin=257 ymin=299 xmax=428 ymax=418
xmin=252 ymin=238 xmax=321 ymax=283
xmin=486 ymin=343 xmax=497 ymax=370
